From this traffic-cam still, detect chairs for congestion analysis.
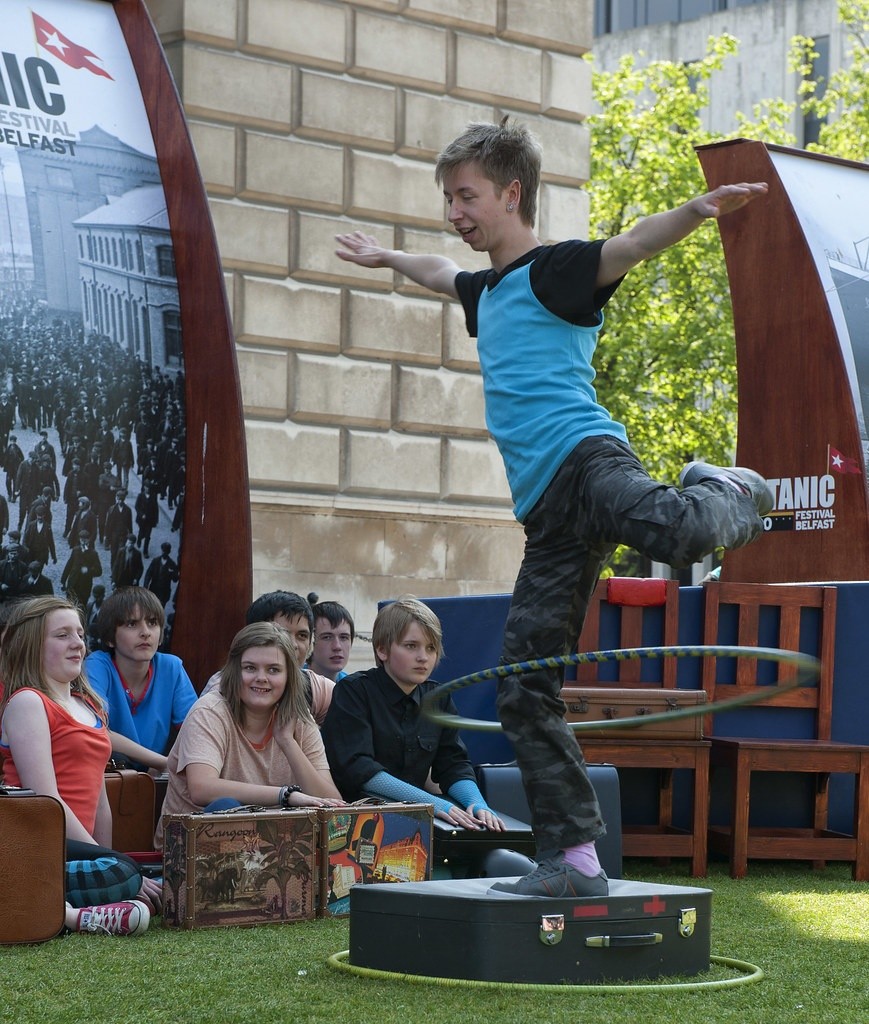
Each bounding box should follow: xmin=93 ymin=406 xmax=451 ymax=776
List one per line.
xmin=700 ymin=581 xmax=869 ymax=882
xmin=573 ymin=577 xmax=714 ymax=876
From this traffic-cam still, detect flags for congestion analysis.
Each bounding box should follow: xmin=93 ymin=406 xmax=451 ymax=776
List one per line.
xmin=35 ymin=12 xmax=116 ymax=83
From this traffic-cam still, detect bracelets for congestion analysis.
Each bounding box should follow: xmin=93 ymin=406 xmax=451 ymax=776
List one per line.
xmin=278 ymin=784 xmax=301 ymax=806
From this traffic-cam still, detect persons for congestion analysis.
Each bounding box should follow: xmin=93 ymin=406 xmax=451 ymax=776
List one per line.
xmin=303 ymin=600 xmax=354 ymax=682
xmin=82 ymin=586 xmax=199 ymax=776
xmin=0 ymin=596 xmax=164 ymax=903
xmin=154 ymin=621 xmax=347 ymax=865
xmin=0 ymin=278 xmax=186 ymax=647
xmin=337 ymin=125 xmax=769 ymax=900
xmin=321 ymin=597 xmax=507 ymax=832
xmin=199 ymin=588 xmax=336 ymax=726
xmin=61 ymin=900 xmax=149 ymax=934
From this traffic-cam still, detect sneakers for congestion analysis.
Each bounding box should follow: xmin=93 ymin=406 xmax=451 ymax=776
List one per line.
xmin=679 ymin=460 xmax=775 ymax=517
xmin=486 ymin=850 xmax=609 ymax=899
xmin=76 ymin=900 xmax=151 ymax=936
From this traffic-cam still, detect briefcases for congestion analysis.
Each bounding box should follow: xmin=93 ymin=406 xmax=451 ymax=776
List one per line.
xmin=163 ymin=796 xmax=434 ymax=932
xmin=102 ymin=759 xmax=157 ymax=852
xmin=479 ymin=756 xmax=623 ymax=879
xmin=0 ymin=786 xmax=66 ymax=945
